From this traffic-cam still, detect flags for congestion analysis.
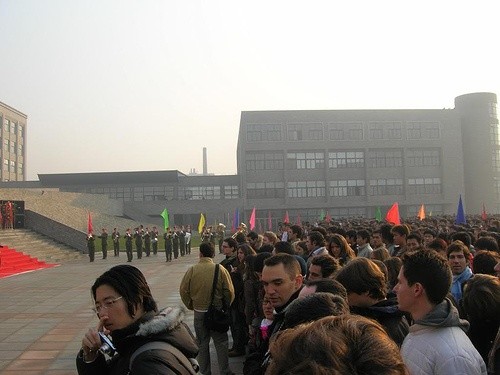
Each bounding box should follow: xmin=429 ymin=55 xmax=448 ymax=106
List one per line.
xmin=88 ymin=212 xmax=92 ymax=233
xmin=367 ymin=202 xmax=400 ymax=225
xmin=198 ymin=213 xmax=205 ymax=233
xmin=455 ymin=195 xmax=466 ymax=223
xmin=418 ymin=204 xmax=433 ymax=219
xmin=482 ymin=203 xmax=487 ymax=221
xmin=161 ymin=208 xmax=169 ymax=230
xmin=231 ymin=208 xmax=330 ymax=232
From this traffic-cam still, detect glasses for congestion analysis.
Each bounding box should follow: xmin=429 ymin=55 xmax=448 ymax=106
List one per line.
xmin=92 ymin=296 xmax=123 ymax=314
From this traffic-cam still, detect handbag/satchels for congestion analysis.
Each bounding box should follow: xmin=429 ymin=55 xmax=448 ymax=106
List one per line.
xmin=206 ymin=304 xmax=230 ymax=332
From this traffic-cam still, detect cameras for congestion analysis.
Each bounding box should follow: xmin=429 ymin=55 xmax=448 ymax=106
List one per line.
xmin=98 ymin=332 xmax=117 ymax=357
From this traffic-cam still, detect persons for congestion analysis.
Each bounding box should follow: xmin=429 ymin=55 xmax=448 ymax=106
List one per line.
xmin=179 ymin=242 xmax=235 ymax=375
xmin=218 ymin=214 xmax=500 ymax=375
xmin=87 ymin=224 xmax=215 ymax=262
xmin=76 ymin=265 xmax=200 ymax=375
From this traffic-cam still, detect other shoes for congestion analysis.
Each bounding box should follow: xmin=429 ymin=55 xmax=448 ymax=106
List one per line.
xmin=228 ymin=348 xmax=245 ymax=356
